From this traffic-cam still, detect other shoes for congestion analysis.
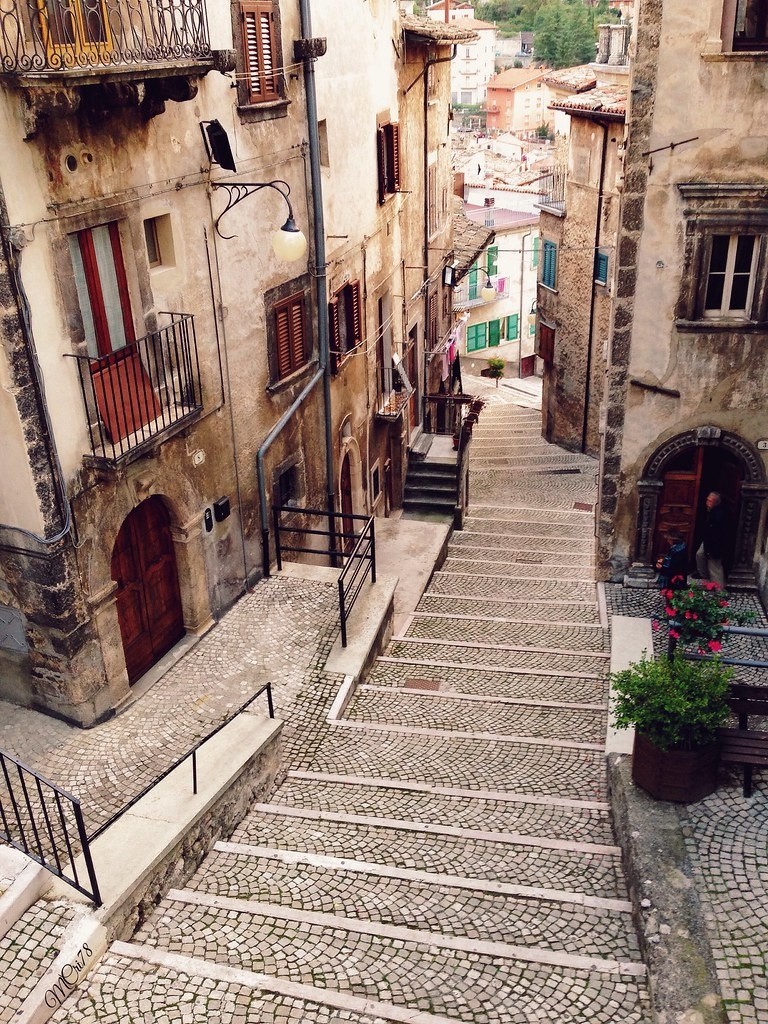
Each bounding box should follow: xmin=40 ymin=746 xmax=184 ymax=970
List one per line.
xmin=692 ymin=573 xmax=702 ymax=579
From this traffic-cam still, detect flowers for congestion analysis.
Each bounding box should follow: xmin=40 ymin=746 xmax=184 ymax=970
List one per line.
xmin=651 ymin=574 xmax=758 ymax=654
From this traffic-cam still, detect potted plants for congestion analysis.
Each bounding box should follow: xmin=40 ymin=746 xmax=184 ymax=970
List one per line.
xmin=602 ymin=642 xmax=740 ymax=805
xmin=451 ymin=398 xmax=485 ymax=447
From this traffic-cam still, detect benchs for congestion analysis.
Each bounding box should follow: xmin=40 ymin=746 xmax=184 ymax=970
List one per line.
xmin=716 ymin=682 xmax=768 ymax=799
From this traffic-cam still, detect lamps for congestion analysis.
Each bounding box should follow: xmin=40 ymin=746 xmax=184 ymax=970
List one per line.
xmin=452 ymin=267 xmax=496 ymax=301
xmin=210 ymin=179 xmax=308 ymax=263
xmin=527 ymin=300 xmax=537 ymax=325
xmin=442 ymin=265 xmax=453 ymax=288
xmin=198 ymin=119 xmax=237 ymax=173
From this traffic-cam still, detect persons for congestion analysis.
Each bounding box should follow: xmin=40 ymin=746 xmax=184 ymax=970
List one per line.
xmin=656 ymin=528 xmax=687 ymax=625
xmin=691 ymin=492 xmax=726 ymax=609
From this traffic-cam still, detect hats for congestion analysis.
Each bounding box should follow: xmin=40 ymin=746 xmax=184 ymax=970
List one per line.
xmin=663 ymin=525 xmax=682 ymax=540
